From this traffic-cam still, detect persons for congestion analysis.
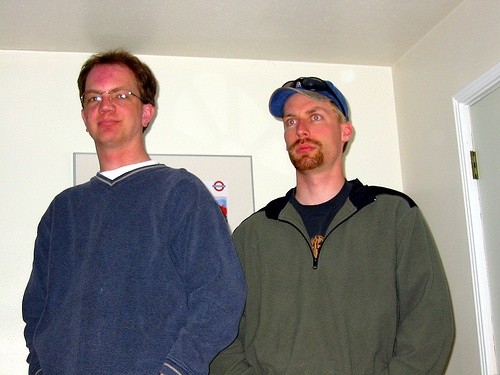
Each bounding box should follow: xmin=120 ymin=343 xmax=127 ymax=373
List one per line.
xmin=22 ymin=50 xmax=247 ymax=375
xmin=209 ymin=77 xmax=456 ymax=375
